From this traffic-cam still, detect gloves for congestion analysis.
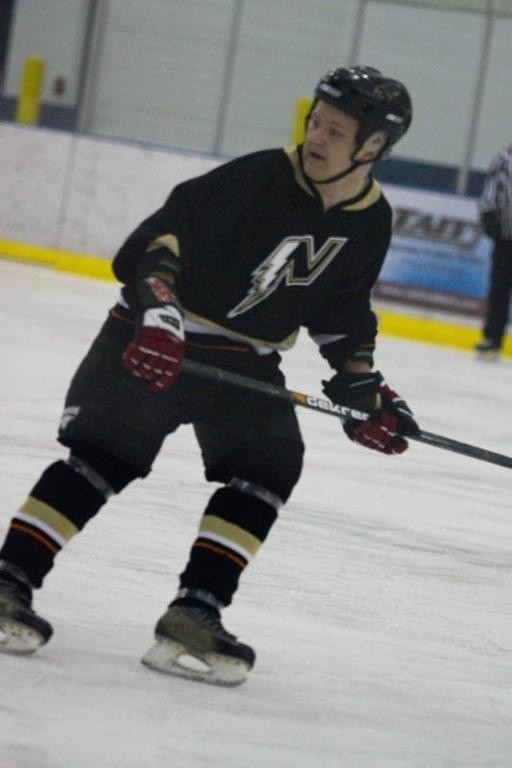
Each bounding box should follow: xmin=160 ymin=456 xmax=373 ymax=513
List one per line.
xmin=481 ymin=210 xmax=501 ymax=241
xmin=124 ymin=274 xmax=188 ymax=396
xmin=323 ymin=369 xmax=418 ymax=455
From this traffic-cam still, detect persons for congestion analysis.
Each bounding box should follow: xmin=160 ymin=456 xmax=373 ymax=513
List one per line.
xmin=2 ymin=63 xmax=422 ymax=668
xmin=473 ymin=142 xmax=510 ymax=353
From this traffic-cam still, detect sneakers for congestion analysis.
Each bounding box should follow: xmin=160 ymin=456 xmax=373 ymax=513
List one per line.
xmin=477 ymin=338 xmax=496 ymax=350
xmin=0 ymin=569 xmax=54 ymax=646
xmin=154 ymin=599 xmax=255 ymax=671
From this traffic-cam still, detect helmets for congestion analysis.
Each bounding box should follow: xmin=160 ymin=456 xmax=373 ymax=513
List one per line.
xmin=303 ymin=65 xmax=414 ymax=164
